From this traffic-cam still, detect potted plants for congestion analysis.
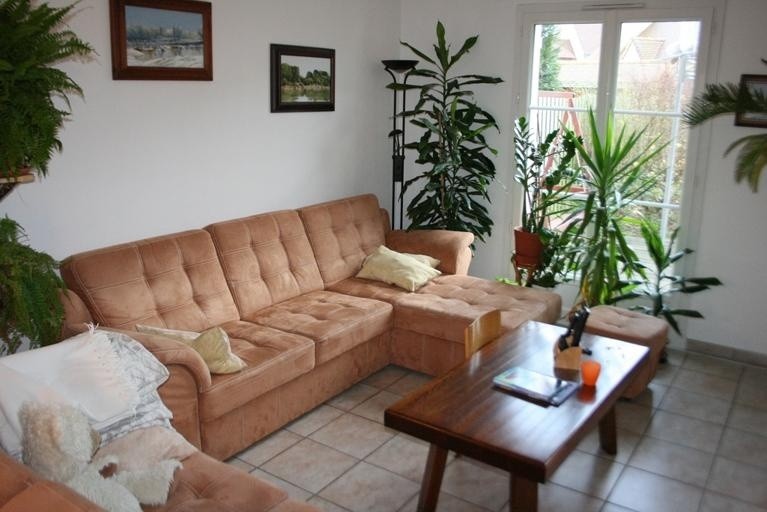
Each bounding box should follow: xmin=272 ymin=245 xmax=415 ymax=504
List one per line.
xmin=514 ymin=111 xmax=583 ymax=266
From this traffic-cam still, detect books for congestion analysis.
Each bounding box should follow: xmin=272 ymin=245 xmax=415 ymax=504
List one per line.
xmin=492 ymin=365 xmax=570 ymax=404
xmin=549 ymin=377 xmax=582 ymax=406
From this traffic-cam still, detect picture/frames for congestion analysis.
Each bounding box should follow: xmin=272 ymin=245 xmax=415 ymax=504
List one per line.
xmin=108 ymin=0 xmax=214 ymax=82
xmin=270 ymin=44 xmax=336 ymax=114
xmin=735 ymin=74 xmax=767 ymax=128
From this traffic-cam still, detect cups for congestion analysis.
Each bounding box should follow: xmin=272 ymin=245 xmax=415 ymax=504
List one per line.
xmin=581 ymin=359 xmax=601 ymax=386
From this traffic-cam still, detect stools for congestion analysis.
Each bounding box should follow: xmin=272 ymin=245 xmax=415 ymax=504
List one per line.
xmin=583 ymin=304 xmax=670 ymax=402
